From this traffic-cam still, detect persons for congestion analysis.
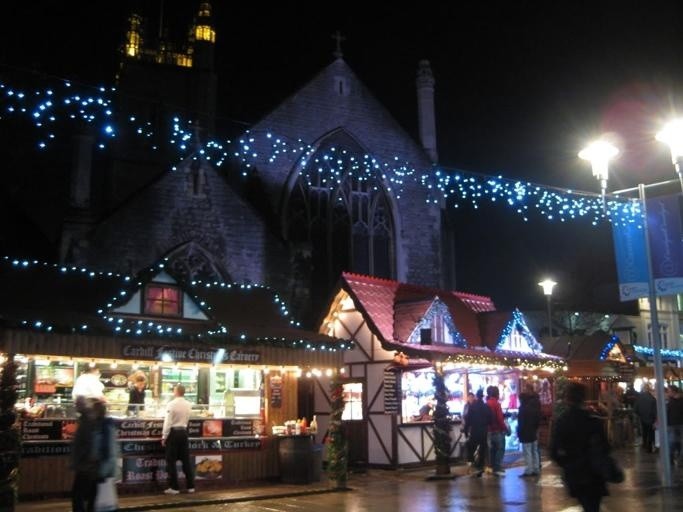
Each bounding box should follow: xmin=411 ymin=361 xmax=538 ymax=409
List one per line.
xmin=624 ymin=382 xmax=683 ymax=469
xmin=71 ymin=365 xmax=105 ymax=401
xmin=462 ymin=383 xmax=542 ymax=477
xmin=549 ymin=383 xmax=624 ymax=512
xmin=69 ymin=395 xmax=122 ymax=512
xmin=161 ymin=384 xmax=195 ymax=494
xmin=126 ymin=372 xmax=148 ymax=415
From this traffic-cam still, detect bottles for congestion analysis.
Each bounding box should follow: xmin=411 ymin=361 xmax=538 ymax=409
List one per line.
xmin=295 ymin=415 xmax=318 ymax=435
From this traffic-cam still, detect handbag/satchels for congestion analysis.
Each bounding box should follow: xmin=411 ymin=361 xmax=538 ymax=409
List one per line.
xmin=600 ymin=444 xmax=627 ymax=486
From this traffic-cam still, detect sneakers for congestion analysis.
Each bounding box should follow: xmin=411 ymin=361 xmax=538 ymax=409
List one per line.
xmin=164 ymin=486 xmax=198 ymax=494
xmin=485 ymin=468 xmax=540 ymax=479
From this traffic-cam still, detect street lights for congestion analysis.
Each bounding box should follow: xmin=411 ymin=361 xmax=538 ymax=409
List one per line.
xmin=577 ymin=117 xmax=682 ymax=487
